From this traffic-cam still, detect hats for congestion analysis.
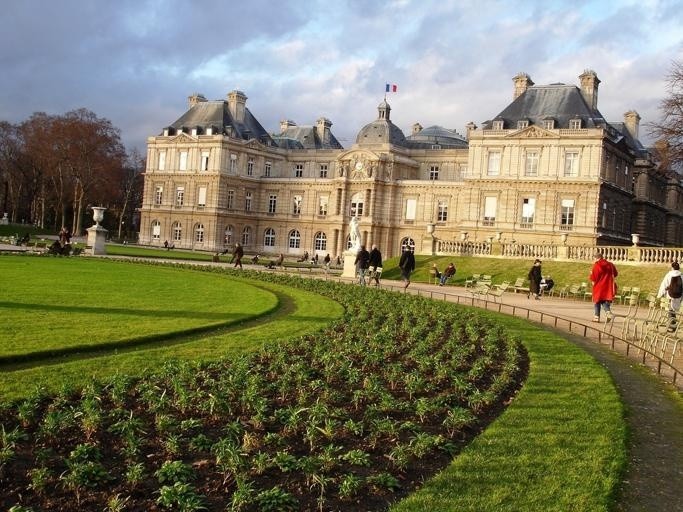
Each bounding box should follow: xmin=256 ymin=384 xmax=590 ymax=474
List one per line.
xmin=534 ymin=259 xmax=542 ymax=264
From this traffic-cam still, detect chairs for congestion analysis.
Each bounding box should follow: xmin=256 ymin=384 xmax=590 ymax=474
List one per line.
xmin=427 ymin=262 xmax=441 ymax=286
xmin=466 ymin=272 xmax=682 ymax=369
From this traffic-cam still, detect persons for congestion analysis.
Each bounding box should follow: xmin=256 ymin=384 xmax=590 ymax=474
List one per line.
xmin=121 ymin=235 xmax=128 ymax=248
xmin=656 ymin=261 xmax=682 ymax=333
xmin=263 ymin=253 xmax=283 ymax=269
xmin=438 ymin=262 xmax=455 ymax=286
xmin=296 ymin=250 xmax=343 ymax=269
xmin=348 ymin=216 xmax=361 ymax=248
xmin=526 ymin=258 xmax=542 ymax=300
xmin=232 ymin=242 xmax=242 ymax=270
xmin=398 ymin=245 xmax=415 ymax=289
xmin=48 ymin=239 xmax=62 ymax=255
xmin=353 ymin=245 xmax=368 ymax=287
xmin=163 ymin=240 xmax=168 ymax=247
xmin=250 ymin=254 xmax=258 ymax=263
xmin=58 ymin=228 xmax=65 ymax=243
xmin=64 ymin=230 xmax=71 ymax=243
xmin=587 ymin=253 xmax=618 ymax=324
xmin=538 ymin=275 xmax=553 ymax=295
xmin=431 ymin=263 xmax=440 ymax=278
xmin=366 ymin=244 xmax=381 ymax=287
xmin=15 ymin=232 xmax=30 ymax=246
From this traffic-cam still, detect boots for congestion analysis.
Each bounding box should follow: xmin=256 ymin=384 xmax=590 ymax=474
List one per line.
xmin=592 ymin=316 xmax=599 ymax=322
xmin=606 ymin=311 xmax=614 ymax=323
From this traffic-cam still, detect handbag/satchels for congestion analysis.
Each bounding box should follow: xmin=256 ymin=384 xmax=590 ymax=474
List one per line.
xmin=613 ymin=281 xmax=617 ymax=296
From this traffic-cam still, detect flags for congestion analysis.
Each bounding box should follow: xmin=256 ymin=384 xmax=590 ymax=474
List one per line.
xmin=385 ymin=84 xmax=396 ymax=93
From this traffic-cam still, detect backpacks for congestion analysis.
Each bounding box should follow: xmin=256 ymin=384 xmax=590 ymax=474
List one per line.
xmin=669 ymin=277 xmax=683 ymax=298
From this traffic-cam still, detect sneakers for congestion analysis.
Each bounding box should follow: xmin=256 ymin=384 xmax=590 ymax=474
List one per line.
xmin=405 ymin=282 xmax=410 ymax=288
